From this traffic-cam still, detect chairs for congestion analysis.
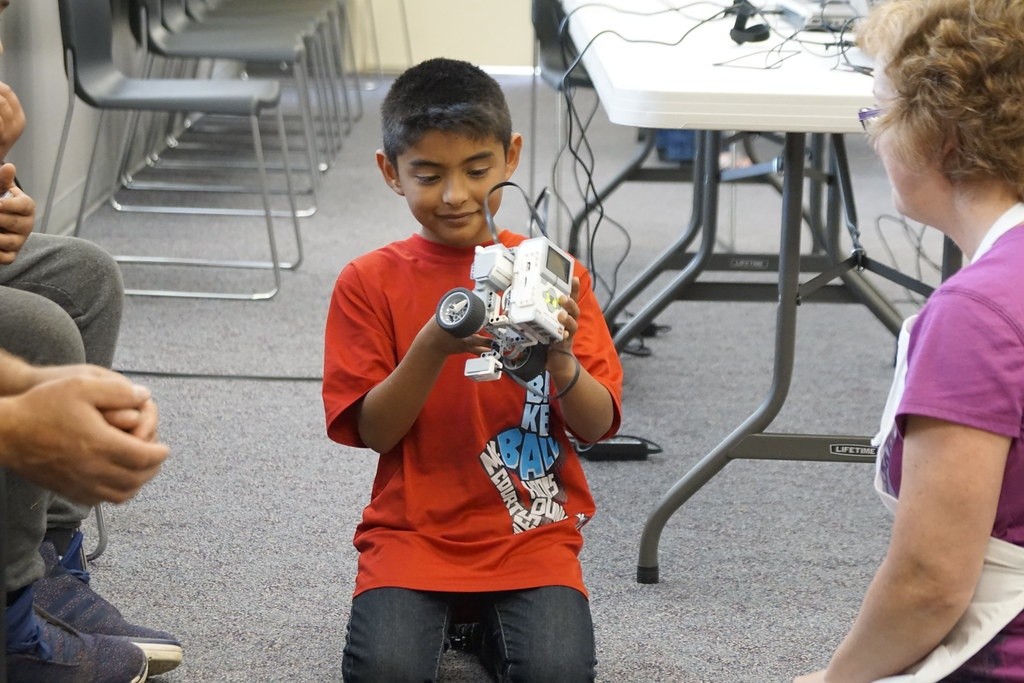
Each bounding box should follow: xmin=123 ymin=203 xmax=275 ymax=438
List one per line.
xmin=530 ymin=1 xmax=737 ymax=272
xmin=38 ymin=0 xmax=304 ymax=301
xmin=109 ymin=0 xmax=382 ymax=219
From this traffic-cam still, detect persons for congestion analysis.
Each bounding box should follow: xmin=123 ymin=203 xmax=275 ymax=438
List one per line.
xmin=322 ymin=57 xmax=625 ymax=683
xmin=788 ymin=0 xmax=1023 ymax=683
xmin=0 ymin=0 xmax=182 ymax=683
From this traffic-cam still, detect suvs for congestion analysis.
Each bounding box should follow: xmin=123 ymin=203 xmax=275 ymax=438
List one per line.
xmin=434 ymin=234 xmax=576 ymax=383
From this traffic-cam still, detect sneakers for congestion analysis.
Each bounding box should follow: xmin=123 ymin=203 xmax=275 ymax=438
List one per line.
xmin=0 ymin=579 xmax=149 ymax=683
xmin=30 ymin=531 xmax=183 ymax=677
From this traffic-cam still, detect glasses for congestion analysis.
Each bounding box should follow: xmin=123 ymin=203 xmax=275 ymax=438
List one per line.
xmin=858 ymin=106 xmax=890 ymax=136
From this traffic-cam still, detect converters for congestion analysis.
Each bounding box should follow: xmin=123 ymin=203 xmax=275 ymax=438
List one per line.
xmin=580 ymin=439 xmax=648 ymax=463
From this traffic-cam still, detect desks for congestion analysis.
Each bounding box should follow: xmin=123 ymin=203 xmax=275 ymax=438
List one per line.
xmin=556 ymin=0 xmax=962 ymax=584
